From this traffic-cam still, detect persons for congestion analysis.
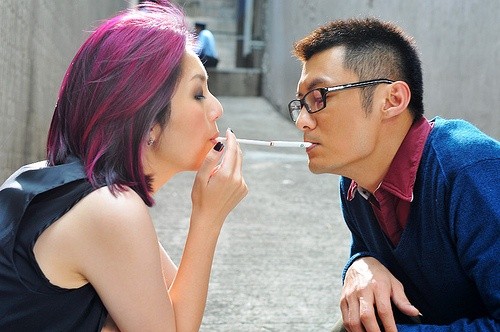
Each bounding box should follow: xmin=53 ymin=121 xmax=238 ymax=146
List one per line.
xmin=192 ymin=21 xmax=219 ymax=68
xmin=0 ymin=0 xmax=250 ymax=332
xmin=289 ymin=18 xmax=499 ymax=332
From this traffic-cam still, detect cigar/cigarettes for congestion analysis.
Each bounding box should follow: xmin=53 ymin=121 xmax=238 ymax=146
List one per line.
xmin=216 ymin=136 xmax=272 ymax=147
xmin=271 ymin=141 xmax=312 ymax=149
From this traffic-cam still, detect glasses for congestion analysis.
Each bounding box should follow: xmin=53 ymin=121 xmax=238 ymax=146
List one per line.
xmin=288 ymin=79 xmax=396 ymax=122
